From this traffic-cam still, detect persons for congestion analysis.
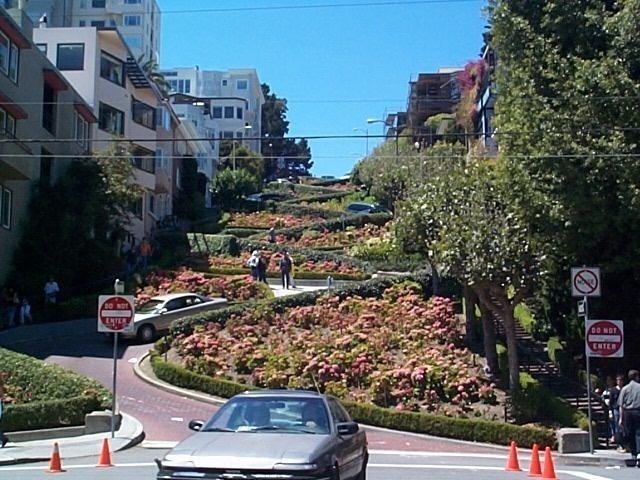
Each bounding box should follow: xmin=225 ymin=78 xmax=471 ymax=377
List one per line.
xmin=247 ymin=249 xmax=295 ymax=290
xmin=594 ymin=369 xmax=640 ymax=468
xmin=126 ymin=237 xmax=160 ymax=281
xmin=302 ymin=403 xmax=325 ymax=429
xmin=5 ymin=278 xmax=60 ymax=327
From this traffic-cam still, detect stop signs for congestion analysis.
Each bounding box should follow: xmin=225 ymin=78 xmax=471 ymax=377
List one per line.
xmin=585 ymin=319 xmax=625 ymax=358
xmin=571 ymin=267 xmax=602 ymax=298
xmin=97 ymin=295 xmax=134 ymax=333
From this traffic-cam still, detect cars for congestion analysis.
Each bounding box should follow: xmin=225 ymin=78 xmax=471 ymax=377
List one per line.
xmin=105 ymin=293 xmax=228 ymax=343
xmin=157 ymin=389 xmax=369 ymax=480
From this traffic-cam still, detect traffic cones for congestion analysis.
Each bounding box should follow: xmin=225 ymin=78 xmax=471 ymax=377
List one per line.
xmin=96 ymin=438 xmax=113 ymax=467
xmin=505 ymin=441 xmax=522 ymax=471
xmin=540 ymin=447 xmax=560 ymax=480
xmin=526 ymin=444 xmax=543 ymax=477
xmin=44 ymin=443 xmax=66 ymax=473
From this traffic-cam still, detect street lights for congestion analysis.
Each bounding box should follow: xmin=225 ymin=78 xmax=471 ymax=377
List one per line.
xmin=232 ymin=126 xmax=252 ymax=170
xmin=353 ymin=128 xmax=368 ymax=156
xmin=367 ymin=118 xmax=398 ymax=165
